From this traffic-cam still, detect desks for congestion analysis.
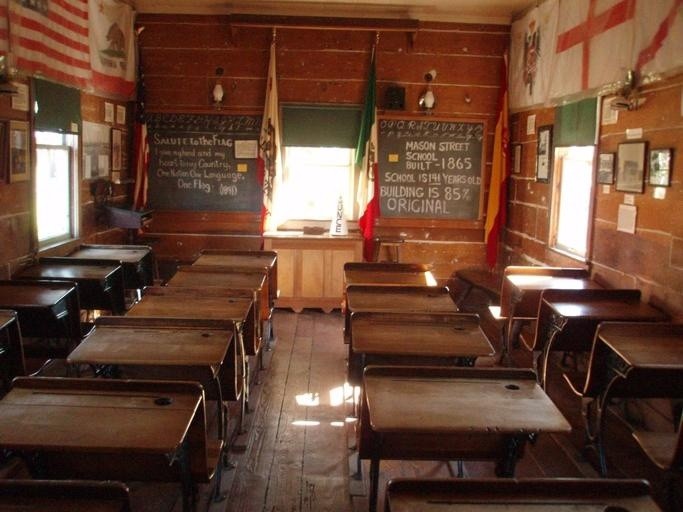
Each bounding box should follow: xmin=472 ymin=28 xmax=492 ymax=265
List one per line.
xmin=0 ymin=240 xmax=278 ymax=512
xmin=344 ymin=261 xmax=683 ymax=512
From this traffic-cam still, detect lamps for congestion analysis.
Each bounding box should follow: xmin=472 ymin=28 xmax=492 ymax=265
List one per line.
xmin=609 ymin=66 xmax=649 ymax=112
xmin=0 ymin=49 xmax=19 ymax=94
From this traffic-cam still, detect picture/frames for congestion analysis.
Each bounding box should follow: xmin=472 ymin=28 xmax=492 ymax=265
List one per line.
xmin=648 ymin=148 xmax=672 ymax=186
xmin=6 ymin=119 xmax=32 ymax=185
xmin=514 ymin=144 xmax=521 ymax=174
xmin=537 ymin=126 xmax=552 ymax=183
xmin=595 ymin=153 xmax=614 ymax=185
xmin=111 ymin=128 xmax=123 ymax=172
xmin=615 ymin=140 xmax=648 ymax=195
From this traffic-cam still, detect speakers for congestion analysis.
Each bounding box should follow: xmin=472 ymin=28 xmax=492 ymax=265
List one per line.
xmin=383 ymin=86 xmax=405 ymax=109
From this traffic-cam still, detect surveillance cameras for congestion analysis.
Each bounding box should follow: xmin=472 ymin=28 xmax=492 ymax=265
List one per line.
xmin=424 ymin=69 xmax=437 ymax=81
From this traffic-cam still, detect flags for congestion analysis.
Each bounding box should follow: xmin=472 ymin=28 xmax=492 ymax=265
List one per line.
xmin=351 ymin=61 xmax=383 ymax=261
xmin=126 ymin=45 xmax=153 ymax=242
xmin=256 ymin=40 xmax=281 ymax=242
xmin=478 ymin=59 xmax=507 ymax=268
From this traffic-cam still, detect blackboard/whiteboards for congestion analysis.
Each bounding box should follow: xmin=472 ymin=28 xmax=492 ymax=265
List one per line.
xmin=378 ymin=114 xmax=488 ymax=230
xmin=141 ymin=109 xmax=262 ymax=214
